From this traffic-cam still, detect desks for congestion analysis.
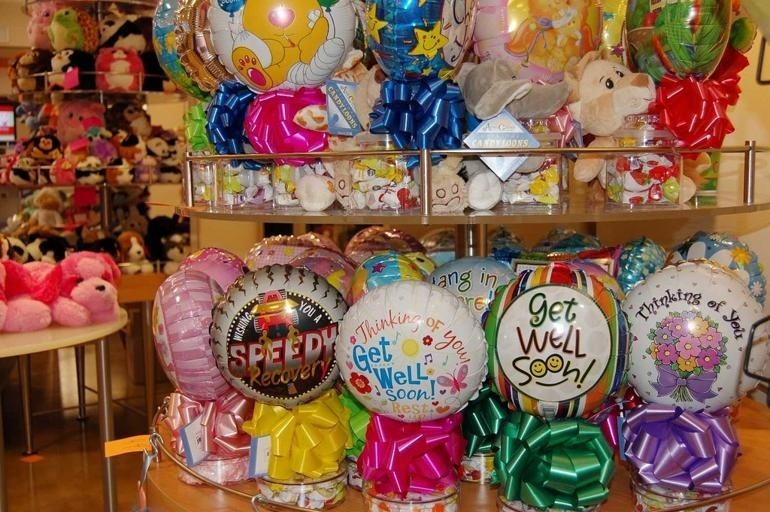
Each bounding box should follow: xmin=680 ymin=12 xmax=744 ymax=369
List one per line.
xmin=0 ymin=308 xmax=128 ymax=512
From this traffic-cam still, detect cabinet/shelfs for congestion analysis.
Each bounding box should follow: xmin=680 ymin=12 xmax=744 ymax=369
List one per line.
xmin=10 ymin=70 xmax=166 ymax=436
xmin=142 ymin=137 xmax=770 ymax=512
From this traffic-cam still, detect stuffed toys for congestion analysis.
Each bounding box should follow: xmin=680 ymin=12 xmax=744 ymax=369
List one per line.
xmin=0 ymin=0 xmax=193 ymax=334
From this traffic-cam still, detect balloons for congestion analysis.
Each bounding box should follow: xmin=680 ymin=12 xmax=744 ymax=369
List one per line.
xmin=152 ymin=0 xmax=770 ymax=511
